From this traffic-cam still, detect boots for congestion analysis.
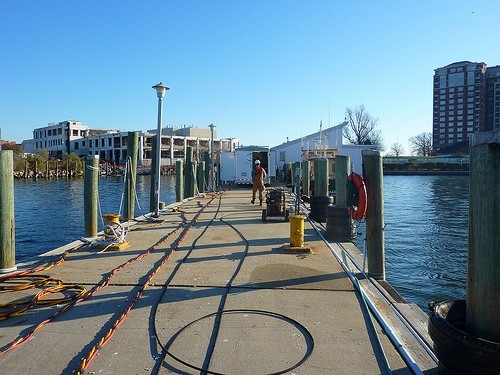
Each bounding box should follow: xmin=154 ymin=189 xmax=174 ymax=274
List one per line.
xmin=251 ymin=199 xmax=255 ymax=204
xmin=260 ymin=199 xmax=262 ymax=206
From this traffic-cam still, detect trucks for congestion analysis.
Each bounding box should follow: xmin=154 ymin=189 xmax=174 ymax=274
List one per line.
xmin=219 ymin=146 xmax=270 ymax=188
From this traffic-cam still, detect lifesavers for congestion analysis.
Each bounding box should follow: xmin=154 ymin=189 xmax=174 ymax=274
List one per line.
xmin=343 ymin=173 xmax=367 ymax=219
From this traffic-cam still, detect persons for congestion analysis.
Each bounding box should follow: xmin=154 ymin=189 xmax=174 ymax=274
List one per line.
xmin=251 ymin=160 xmax=266 ymax=205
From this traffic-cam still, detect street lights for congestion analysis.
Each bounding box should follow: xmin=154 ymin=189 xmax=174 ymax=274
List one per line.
xmin=208 ymin=122 xmax=216 ymax=192
xmin=151 ymin=82 xmax=170 ymax=216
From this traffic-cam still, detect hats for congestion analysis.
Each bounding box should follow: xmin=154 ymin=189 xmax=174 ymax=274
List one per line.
xmin=255 ymin=160 xmax=260 ymax=164
